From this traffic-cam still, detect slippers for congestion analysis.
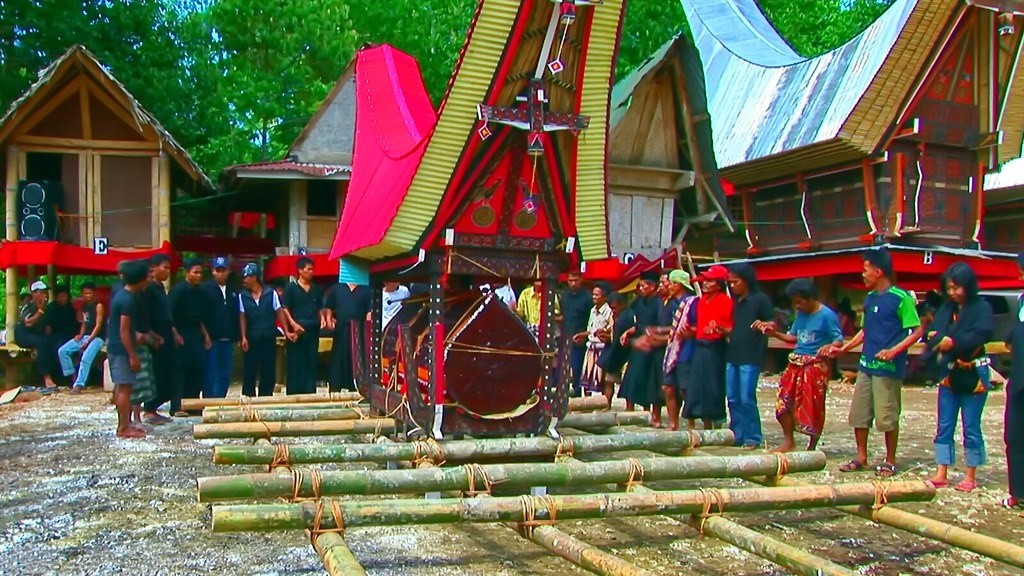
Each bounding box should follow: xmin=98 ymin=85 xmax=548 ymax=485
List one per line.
xmin=875 ymin=463 xmax=897 ymax=477
xmin=839 ymin=459 xmax=870 ymax=472
xmin=954 ymin=481 xmax=975 ymax=492
xmin=143 ymin=413 xmax=174 ymax=425
xmin=925 ymin=479 xmax=950 ymax=488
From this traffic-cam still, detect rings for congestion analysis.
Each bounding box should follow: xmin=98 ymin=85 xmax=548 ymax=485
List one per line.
xmin=882 ymin=356 xmax=886 ymax=359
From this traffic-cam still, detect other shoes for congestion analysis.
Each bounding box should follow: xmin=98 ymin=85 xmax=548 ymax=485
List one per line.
xmin=69 ymin=389 xmax=81 ymax=396
xmin=21 ymin=385 xmax=36 ymax=392
xmin=40 ymin=385 xmax=60 ymax=395
xmin=996 ymin=497 xmax=1017 ymax=507
xmin=743 ymin=445 xmax=758 ymax=450
xmin=768 ymin=446 xmax=795 ymax=453
xmin=175 ymin=410 xmax=190 ymax=417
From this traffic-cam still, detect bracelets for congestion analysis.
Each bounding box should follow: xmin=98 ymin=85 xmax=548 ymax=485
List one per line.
xmin=38 ymin=308 xmax=45 ymax=315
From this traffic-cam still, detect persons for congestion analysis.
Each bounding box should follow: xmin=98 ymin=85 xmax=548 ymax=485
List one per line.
xmin=16 ymin=255 xmax=412 ymax=440
xmin=485 ymin=250 xmax=1024 ymax=508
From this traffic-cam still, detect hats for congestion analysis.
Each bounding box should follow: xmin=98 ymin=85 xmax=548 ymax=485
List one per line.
xmin=240 ymin=263 xmax=259 ymax=278
xmin=669 ymin=269 xmax=694 ymax=292
xmin=700 ymin=266 xmax=728 ymax=282
xmin=31 ymin=281 xmax=51 ymax=291
xmin=640 ymin=270 xmax=661 ymax=281
xmin=212 ymin=256 xmax=229 ymax=269
xmin=730 ymin=263 xmax=755 ymax=288
